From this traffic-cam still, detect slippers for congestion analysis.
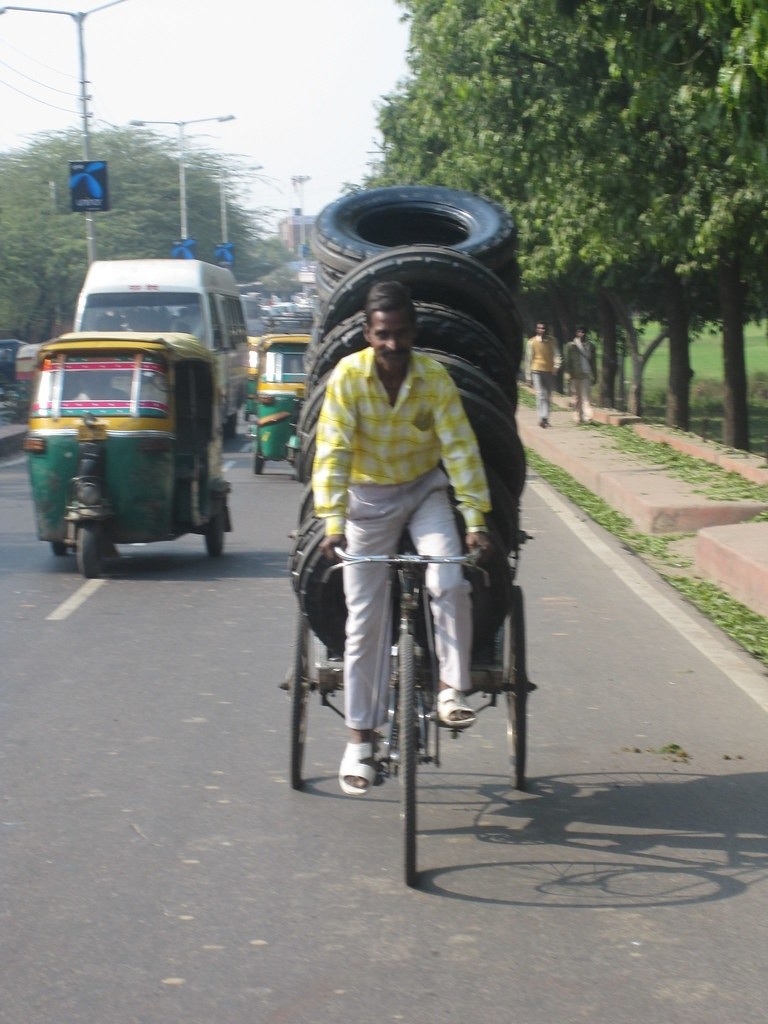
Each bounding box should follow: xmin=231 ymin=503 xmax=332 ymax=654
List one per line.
xmin=436 ymin=688 xmax=477 ymax=727
xmin=338 ymin=741 xmax=377 ymax=795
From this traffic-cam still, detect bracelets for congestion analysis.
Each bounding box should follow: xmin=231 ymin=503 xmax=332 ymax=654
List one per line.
xmin=465 ymin=526 xmax=488 ymax=533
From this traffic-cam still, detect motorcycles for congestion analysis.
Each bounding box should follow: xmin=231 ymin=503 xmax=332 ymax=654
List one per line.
xmin=16 ymin=333 xmax=232 ymax=582
xmin=236 ymin=336 xmax=268 ymax=420
xmin=254 ymin=333 xmax=318 ymax=476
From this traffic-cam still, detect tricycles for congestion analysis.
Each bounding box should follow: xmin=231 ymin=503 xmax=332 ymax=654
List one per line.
xmin=276 ymin=548 xmax=539 ymax=885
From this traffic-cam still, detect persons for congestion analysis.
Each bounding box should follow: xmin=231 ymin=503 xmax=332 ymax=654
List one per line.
xmin=312 ymin=280 xmax=493 ymax=796
xmin=564 ymin=327 xmax=598 ymax=424
xmin=524 ymin=321 xmax=563 ymax=428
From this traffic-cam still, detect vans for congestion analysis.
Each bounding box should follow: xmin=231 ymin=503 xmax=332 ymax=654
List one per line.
xmin=73 ymin=258 xmax=249 ymax=437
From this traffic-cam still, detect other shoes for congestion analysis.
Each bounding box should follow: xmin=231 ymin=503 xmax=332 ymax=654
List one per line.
xmin=539 ymin=417 xmax=549 ymax=427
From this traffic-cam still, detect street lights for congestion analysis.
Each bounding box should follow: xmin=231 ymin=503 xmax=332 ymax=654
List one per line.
xmin=215 ymin=164 xmax=264 ymax=270
xmin=128 ymin=115 xmax=237 ymax=257
xmin=0 ymin=0 xmax=128 ymax=265
